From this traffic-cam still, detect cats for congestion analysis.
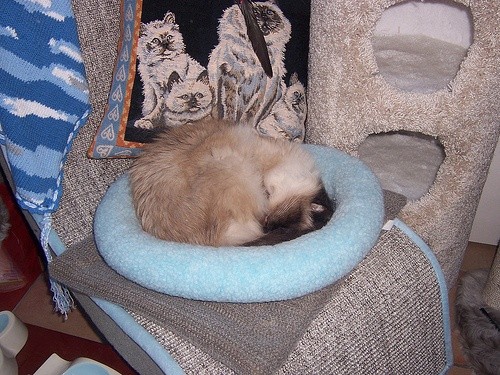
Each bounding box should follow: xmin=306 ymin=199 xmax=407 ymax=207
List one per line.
xmin=121 ymin=116 xmax=327 ymax=246
